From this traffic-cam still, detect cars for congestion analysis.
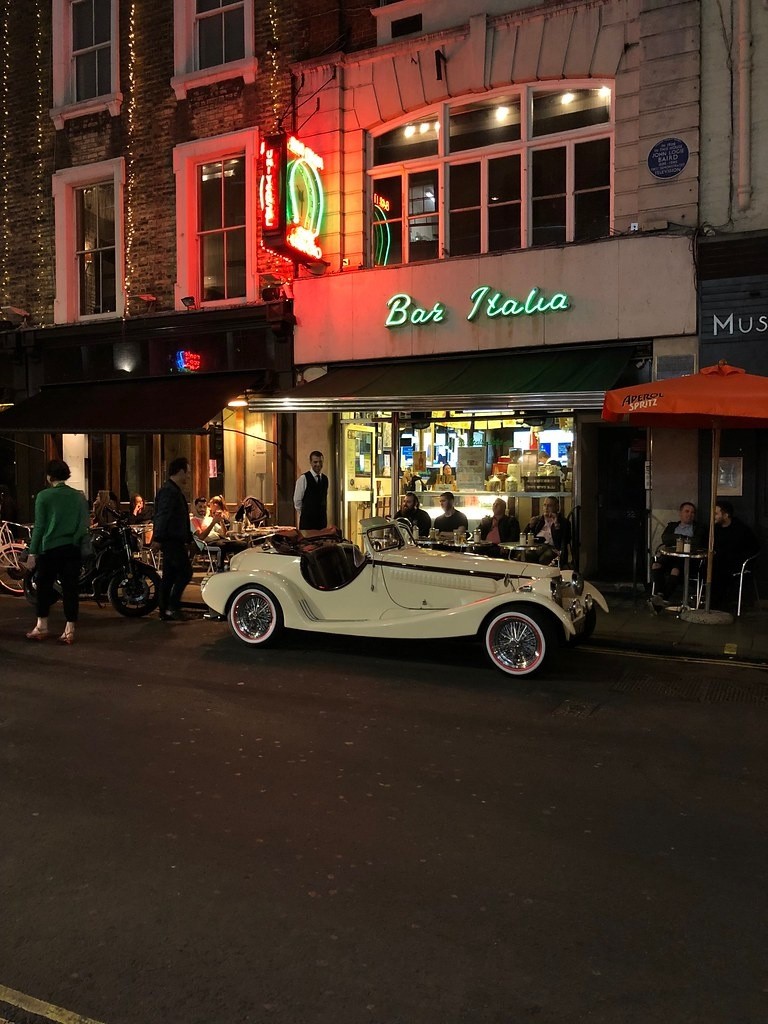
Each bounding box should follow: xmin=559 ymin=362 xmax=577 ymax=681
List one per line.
xmin=201 ymin=516 xmax=612 ymax=678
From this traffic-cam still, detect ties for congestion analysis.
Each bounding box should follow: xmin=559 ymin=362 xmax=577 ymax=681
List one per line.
xmin=317 ymin=475 xmax=321 ymax=483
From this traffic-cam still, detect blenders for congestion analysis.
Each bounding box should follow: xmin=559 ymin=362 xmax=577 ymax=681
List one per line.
xmin=507 ymin=448 xmax=521 ymax=484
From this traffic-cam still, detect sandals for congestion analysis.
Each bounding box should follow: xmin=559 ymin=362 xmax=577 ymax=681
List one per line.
xmin=57 ymin=631 xmax=75 ymax=645
xmin=26 ymin=625 xmax=49 ymax=640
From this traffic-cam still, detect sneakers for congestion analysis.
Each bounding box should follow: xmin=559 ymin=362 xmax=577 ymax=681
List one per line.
xmin=646 ymin=594 xmax=671 ymax=616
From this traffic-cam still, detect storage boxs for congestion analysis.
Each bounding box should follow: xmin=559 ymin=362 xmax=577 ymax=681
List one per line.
xmin=520 ymin=476 xmax=561 ymax=492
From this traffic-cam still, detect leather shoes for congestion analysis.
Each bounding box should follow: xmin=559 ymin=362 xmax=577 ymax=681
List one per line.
xmin=159 ymin=608 xmax=190 ymax=622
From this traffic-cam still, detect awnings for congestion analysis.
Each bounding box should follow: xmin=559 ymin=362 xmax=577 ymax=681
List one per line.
xmin=0 ymin=370 xmax=269 ymax=433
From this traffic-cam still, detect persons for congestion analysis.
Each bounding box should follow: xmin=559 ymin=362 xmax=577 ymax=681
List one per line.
xmin=474 ymin=498 xmax=520 ymax=543
xmin=701 ymin=500 xmax=757 ymax=618
xmin=26 ymin=459 xmax=91 ymax=644
xmin=539 ymin=452 xmax=564 ymax=471
xmin=191 ymin=493 xmax=247 ymax=573
xmin=386 ymin=492 xmax=431 ymax=538
xmin=522 ymin=496 xmax=573 ymax=565
xmin=78 ymin=490 xmax=161 ymax=564
xmin=403 ymin=463 xmax=457 ymax=491
xmin=152 ymin=458 xmax=194 ymax=622
xmin=657 ymin=501 xmax=706 ymax=603
xmin=294 ymin=451 xmax=329 ymax=530
xmin=432 ymin=493 xmax=468 ymax=536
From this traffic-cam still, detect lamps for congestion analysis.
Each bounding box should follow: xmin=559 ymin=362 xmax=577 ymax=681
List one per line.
xmin=129 ymin=294 xmax=156 ymax=313
xmin=180 ymin=296 xmax=196 ymax=307
xmin=262 ymin=288 xmax=280 ymax=301
xmin=1 ymin=306 xmax=29 ymax=329
xmin=229 ymin=395 xmax=250 ymax=407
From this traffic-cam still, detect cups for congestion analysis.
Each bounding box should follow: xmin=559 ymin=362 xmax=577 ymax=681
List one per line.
xmin=684 ymin=539 xmax=690 ymax=553
xmin=506 ymin=481 xmax=518 ymax=492
xmin=676 ymin=539 xmax=684 ymax=553
xmin=412 ymin=525 xmax=419 ymax=540
xmin=474 ymin=529 xmax=481 ymax=543
xmin=520 ymin=533 xmax=526 ymax=545
xmin=453 ymin=530 xmax=461 ymax=544
xmin=427 ymin=486 xmax=432 ymax=490
xmin=461 ymin=533 xmax=466 ymax=544
xmin=527 ymin=533 xmax=534 ymax=546
xmin=435 ymin=529 xmax=440 ymax=541
xmin=430 ymin=528 xmax=435 ymax=541
xmin=487 ymin=481 xmax=501 ymax=491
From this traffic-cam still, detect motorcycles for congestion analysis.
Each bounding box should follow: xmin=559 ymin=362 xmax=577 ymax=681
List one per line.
xmin=0 ymin=520 xmax=35 ymax=596
xmin=22 ymin=505 xmax=162 ymax=617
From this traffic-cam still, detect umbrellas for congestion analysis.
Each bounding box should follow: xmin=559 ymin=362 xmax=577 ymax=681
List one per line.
xmin=602 ymin=360 xmax=768 ymax=624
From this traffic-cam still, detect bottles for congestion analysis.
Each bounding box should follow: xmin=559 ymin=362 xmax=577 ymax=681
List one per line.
xmin=565 ymin=469 xmax=573 ymax=492
xmin=418 ymin=452 xmax=423 ymax=467
xmin=453 ymin=483 xmax=456 ymax=491
xmin=415 ymin=480 xmax=422 ymax=492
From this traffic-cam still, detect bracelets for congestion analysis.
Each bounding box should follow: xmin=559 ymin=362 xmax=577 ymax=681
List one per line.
xmin=28 ymin=554 xmax=36 ymax=557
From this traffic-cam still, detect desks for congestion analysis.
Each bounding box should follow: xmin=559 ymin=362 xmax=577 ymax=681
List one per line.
xmin=416 ymin=536 xmax=453 ymax=550
xmin=660 ymin=545 xmax=720 ymax=610
xmin=498 ymin=542 xmax=543 ymax=562
xmin=444 ymin=540 xmax=493 ymax=554
xmin=227 ymin=525 xmax=296 ymax=537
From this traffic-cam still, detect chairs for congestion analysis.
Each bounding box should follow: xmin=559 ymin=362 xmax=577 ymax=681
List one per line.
xmin=132 ymin=526 xmax=221 ymax=574
xmin=648 ymin=548 xmax=699 ymax=610
xmin=697 ymin=553 xmax=759 ymax=616
